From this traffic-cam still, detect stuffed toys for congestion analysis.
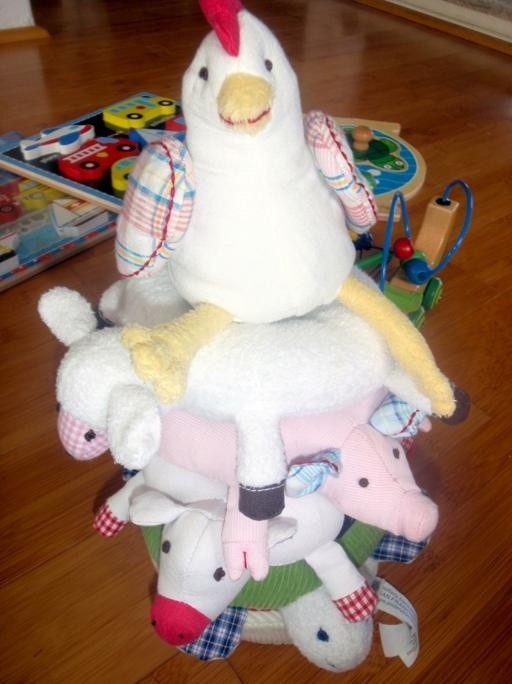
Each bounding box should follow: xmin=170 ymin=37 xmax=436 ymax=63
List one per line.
xmin=36 ymin=1 xmax=472 ymax=673
xmin=114 ymin=0 xmax=378 ymax=322
xmin=40 ymin=270 xmax=457 ymax=674
